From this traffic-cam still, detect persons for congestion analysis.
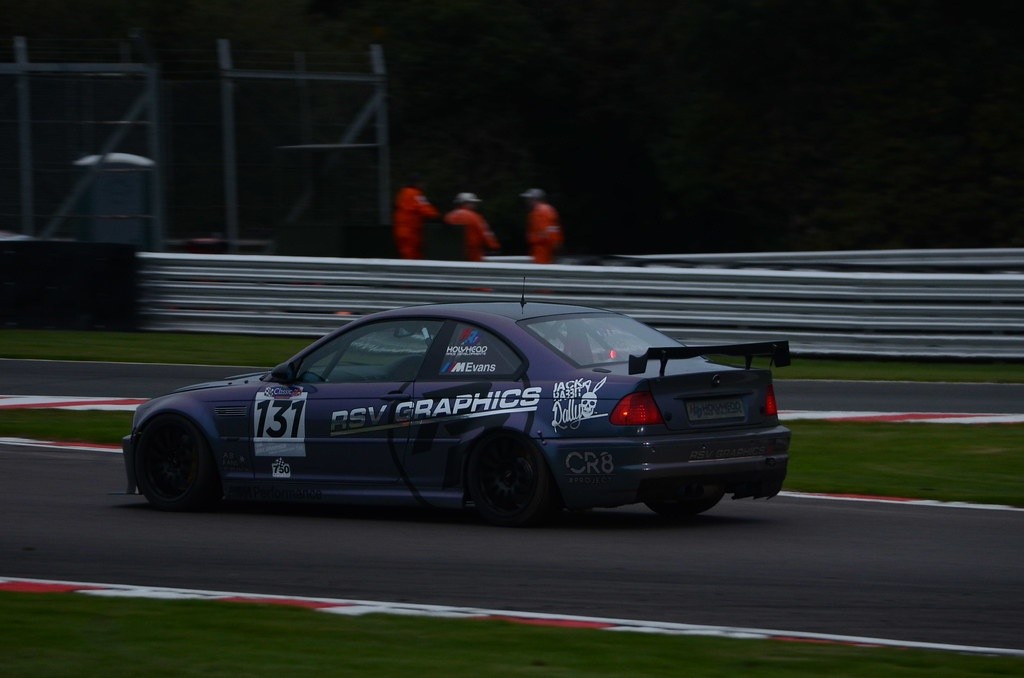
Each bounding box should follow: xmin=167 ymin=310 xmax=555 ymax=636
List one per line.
xmin=394 ymin=172 xmax=439 ymax=261
xmin=520 ymin=188 xmax=562 ymax=294
xmin=443 ymin=193 xmax=498 ymax=293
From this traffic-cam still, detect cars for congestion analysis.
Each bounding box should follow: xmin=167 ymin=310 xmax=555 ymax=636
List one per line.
xmin=120 ymin=296 xmax=793 ymax=521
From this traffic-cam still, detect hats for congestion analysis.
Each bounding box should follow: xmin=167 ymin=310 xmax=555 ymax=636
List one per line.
xmin=455 ymin=192 xmax=483 ymax=203
xmin=519 ymin=189 xmax=546 ymax=198
